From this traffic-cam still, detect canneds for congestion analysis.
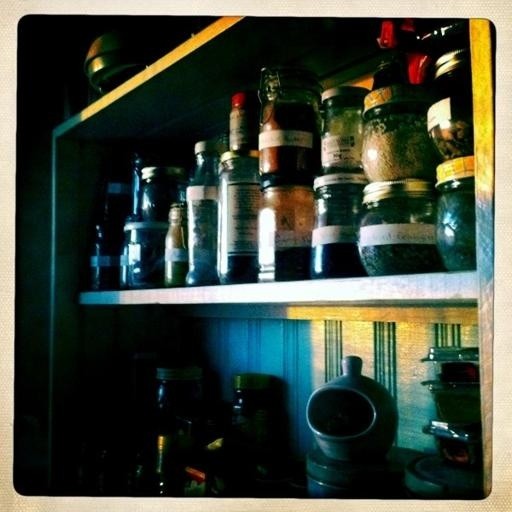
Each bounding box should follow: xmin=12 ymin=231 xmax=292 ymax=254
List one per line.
xmin=256 ymin=68 xmax=320 ymax=182
xmin=117 ymin=219 xmax=161 ymax=290
xmin=254 ymin=173 xmax=314 ymax=282
xmin=212 ymin=153 xmax=257 ymax=283
xmin=137 ymin=164 xmax=180 ymax=219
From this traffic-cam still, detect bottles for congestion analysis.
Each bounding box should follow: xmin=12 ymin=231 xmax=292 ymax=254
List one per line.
xmin=229 ymin=371 xmax=274 ymax=438
xmin=166 ymin=201 xmax=187 ymax=287
xmin=186 ymin=140 xmax=225 ymax=284
xmin=219 ymin=90 xmax=258 ymax=280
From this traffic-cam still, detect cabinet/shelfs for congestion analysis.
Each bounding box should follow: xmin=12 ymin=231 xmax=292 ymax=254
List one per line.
xmin=45 ymin=16 xmax=493 ymax=497
